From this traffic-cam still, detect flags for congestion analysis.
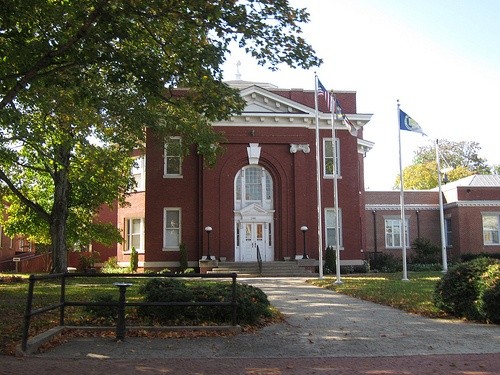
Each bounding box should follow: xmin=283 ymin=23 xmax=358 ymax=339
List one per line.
xmin=342 ymin=114 xmax=353 ymax=128
xmin=336 ymin=100 xmax=343 ymax=120
xmin=400 ymin=110 xmax=428 ymax=138
xmin=318 ymin=79 xmax=328 ymax=98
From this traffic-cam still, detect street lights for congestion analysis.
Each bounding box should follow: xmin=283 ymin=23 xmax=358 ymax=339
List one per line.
xmin=300 ymin=225 xmax=309 ymax=259
xmin=204 ymin=225 xmax=213 ymax=260
